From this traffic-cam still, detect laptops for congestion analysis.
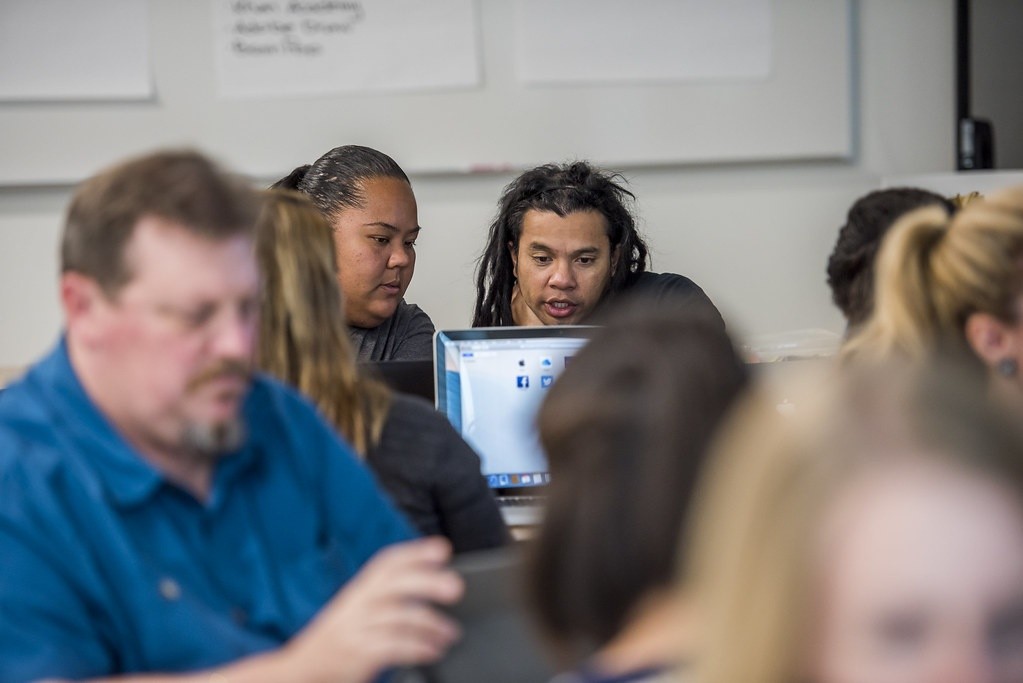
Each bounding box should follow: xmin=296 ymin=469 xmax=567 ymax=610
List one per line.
xmin=432 ymin=324 xmax=595 ymax=526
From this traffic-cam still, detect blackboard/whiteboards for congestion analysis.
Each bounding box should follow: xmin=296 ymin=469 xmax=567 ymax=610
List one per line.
xmin=0 ymin=0 xmax=857 ymax=187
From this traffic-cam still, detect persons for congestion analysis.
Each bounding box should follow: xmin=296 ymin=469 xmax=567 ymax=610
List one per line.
xmin=1 ymin=149 xmax=469 ymax=683
xmin=259 ymin=187 xmax=515 ymax=557
xmin=268 ymin=145 xmax=438 ymax=360
xmin=526 ymin=182 xmax=1023 ymax=683
xmin=474 ymin=162 xmax=727 ymax=330
